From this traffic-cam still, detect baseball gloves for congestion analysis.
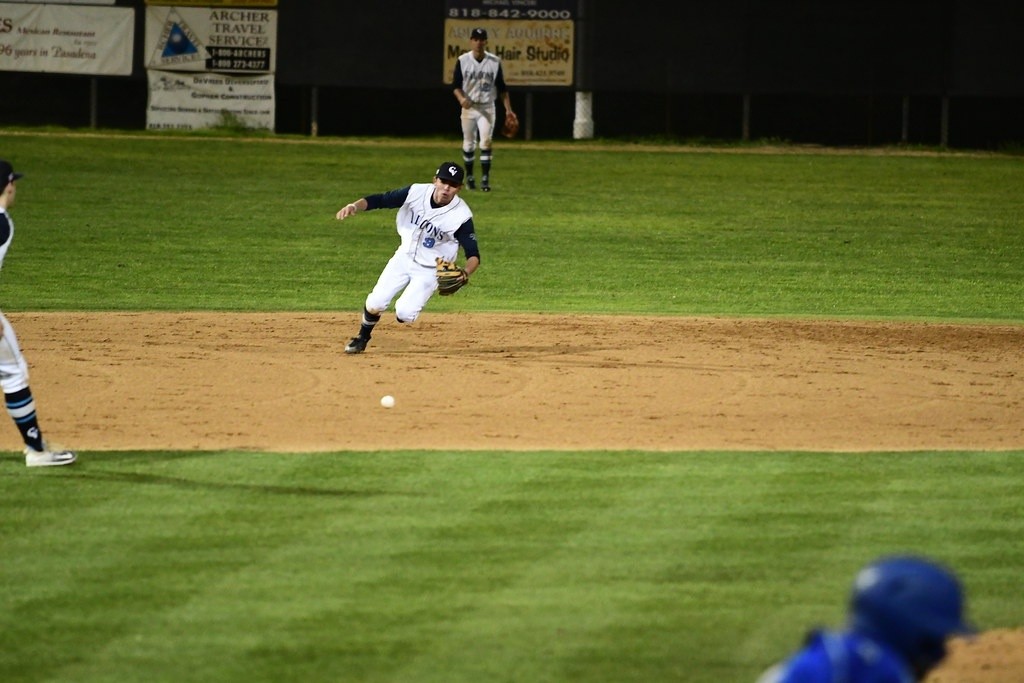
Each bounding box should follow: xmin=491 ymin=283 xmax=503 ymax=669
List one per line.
xmin=435 ymin=257 xmax=469 ymax=296
xmin=502 ymin=116 xmax=518 ymax=139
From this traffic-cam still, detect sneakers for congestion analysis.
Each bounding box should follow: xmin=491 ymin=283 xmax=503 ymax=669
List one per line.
xmin=345 ymin=334 xmax=372 ymax=353
xmin=24 ymin=442 xmax=77 ymax=466
xmin=397 ymin=317 xmax=404 ymax=323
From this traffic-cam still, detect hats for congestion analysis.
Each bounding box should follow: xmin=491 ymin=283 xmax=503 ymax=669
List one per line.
xmin=470 ymin=28 xmax=488 ymax=40
xmin=436 ymin=162 xmax=464 ymax=185
xmin=0 ymin=161 xmax=23 ymax=185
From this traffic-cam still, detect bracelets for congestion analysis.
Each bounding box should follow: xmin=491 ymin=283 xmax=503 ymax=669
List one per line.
xmin=346 ymin=204 xmax=358 ymax=213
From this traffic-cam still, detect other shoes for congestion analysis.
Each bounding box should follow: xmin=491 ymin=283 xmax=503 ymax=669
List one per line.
xmin=466 ymin=176 xmax=476 ymax=190
xmin=481 ymin=181 xmax=491 ymax=192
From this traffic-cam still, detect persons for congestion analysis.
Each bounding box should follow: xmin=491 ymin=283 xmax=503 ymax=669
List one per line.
xmin=451 ymin=28 xmax=516 ymax=191
xmin=0 ymin=160 xmax=78 ymax=467
xmin=334 ymin=161 xmax=480 ymax=354
xmin=749 ymin=553 xmax=977 ymax=683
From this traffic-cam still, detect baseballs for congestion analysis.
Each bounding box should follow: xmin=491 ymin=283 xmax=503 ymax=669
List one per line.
xmin=381 ymin=395 xmax=394 ymax=408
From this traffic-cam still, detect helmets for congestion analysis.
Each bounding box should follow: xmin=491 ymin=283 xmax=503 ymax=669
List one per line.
xmin=850 ymin=559 xmax=981 ymax=671
